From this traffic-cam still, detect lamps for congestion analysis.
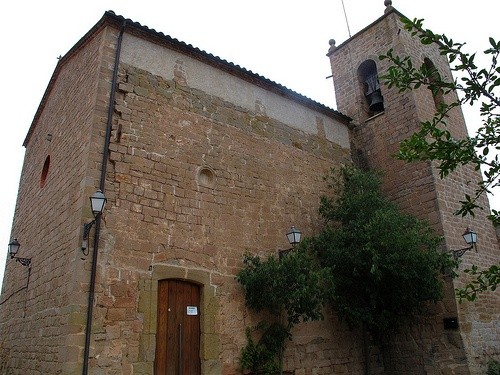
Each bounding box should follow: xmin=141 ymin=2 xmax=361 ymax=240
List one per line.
xmin=8 ymin=237 xmax=32 ymax=267
xmin=449 ymin=226 xmax=478 ymax=260
xmin=278 ymin=225 xmax=302 ymax=260
xmin=81 ymin=190 xmax=108 ymax=255
